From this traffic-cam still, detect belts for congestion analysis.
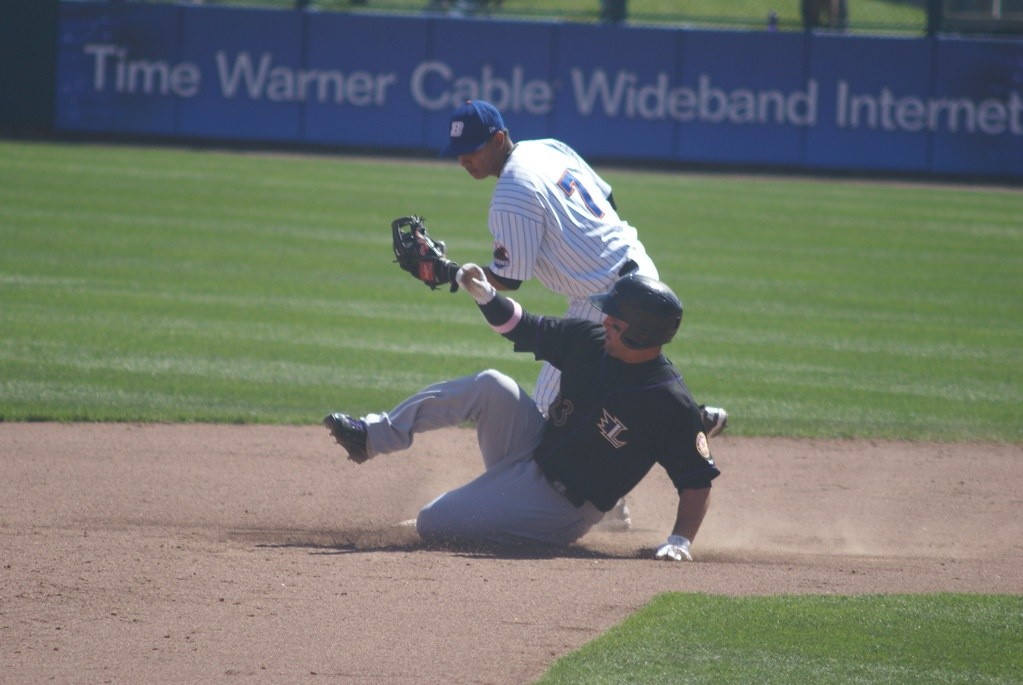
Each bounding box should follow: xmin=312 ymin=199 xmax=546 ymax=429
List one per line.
xmin=616 ymin=260 xmax=638 ymax=278
xmin=534 ymin=443 xmax=584 ymax=508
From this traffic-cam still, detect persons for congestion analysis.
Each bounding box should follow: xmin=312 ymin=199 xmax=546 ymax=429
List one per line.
xmin=323 ymin=263 xmax=721 ymax=561
xmin=391 ymin=99 xmax=728 ymax=438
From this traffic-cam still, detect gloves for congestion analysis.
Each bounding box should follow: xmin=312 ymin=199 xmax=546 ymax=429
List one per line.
xmin=656 ymin=535 xmax=692 ymax=562
xmin=455 ymin=262 xmax=497 ymax=305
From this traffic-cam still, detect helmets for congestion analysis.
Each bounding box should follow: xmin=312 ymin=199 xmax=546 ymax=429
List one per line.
xmin=588 ymin=274 xmax=684 ymax=350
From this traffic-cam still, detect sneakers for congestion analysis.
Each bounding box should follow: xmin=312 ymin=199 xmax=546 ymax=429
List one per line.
xmin=699 ymin=404 xmax=728 ymax=441
xmin=324 ymin=412 xmax=371 ymax=465
xmin=596 ymin=498 xmax=633 ymax=532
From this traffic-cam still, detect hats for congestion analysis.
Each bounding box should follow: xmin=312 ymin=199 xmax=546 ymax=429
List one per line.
xmin=437 ymin=100 xmax=505 ymax=157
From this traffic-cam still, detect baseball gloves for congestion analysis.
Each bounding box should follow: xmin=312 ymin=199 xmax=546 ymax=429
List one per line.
xmin=391 ymin=214 xmax=462 ymax=294
